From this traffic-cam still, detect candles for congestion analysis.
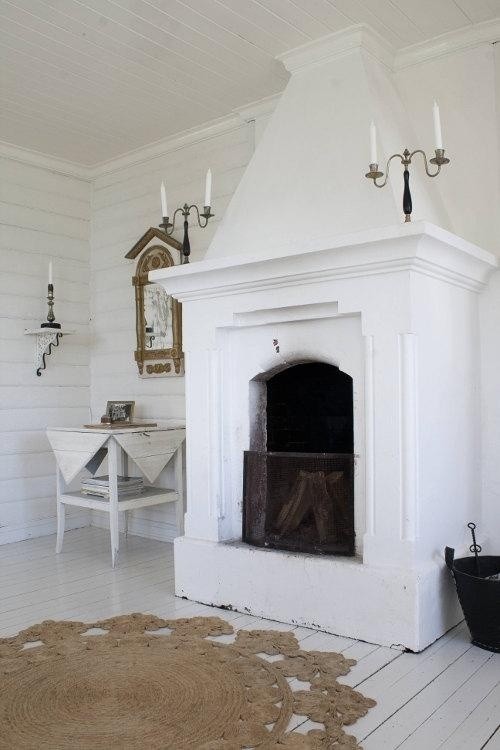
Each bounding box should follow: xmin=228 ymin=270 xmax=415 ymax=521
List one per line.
xmin=48 ymin=256 xmax=53 ymax=283
xmin=370 ymin=121 xmax=377 ymax=163
xmin=204 ymin=168 xmax=212 ymax=206
xmin=432 ymin=98 xmax=442 ymax=149
xmin=160 ymin=180 xmax=168 ymax=217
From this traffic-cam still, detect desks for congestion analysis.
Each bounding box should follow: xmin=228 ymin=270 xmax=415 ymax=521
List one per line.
xmin=47 ymin=426 xmax=187 ymax=568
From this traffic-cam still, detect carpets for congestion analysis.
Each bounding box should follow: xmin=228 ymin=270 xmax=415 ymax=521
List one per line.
xmin=0 ymin=612 xmax=376 ymax=750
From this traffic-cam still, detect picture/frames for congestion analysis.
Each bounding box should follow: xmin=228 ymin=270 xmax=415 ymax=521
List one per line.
xmin=105 ymin=401 xmax=135 ymax=426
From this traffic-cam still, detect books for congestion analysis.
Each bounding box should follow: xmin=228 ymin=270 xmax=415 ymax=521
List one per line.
xmin=83 ymin=476 xmax=144 ymax=498
